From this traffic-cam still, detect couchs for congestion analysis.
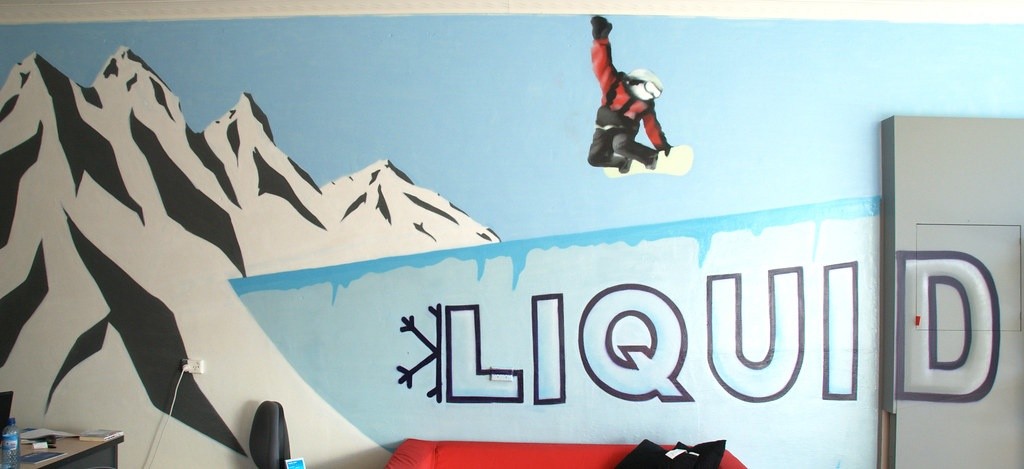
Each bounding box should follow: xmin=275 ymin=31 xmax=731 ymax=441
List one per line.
xmin=383 ymin=437 xmax=748 ymax=469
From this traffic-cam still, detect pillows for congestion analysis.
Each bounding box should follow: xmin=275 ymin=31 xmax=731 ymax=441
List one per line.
xmin=673 ymin=440 xmax=727 ymax=469
xmin=614 ymin=438 xmax=700 ymax=469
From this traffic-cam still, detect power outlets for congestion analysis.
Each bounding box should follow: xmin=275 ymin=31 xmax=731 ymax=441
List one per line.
xmin=188 ymin=359 xmax=204 ymax=374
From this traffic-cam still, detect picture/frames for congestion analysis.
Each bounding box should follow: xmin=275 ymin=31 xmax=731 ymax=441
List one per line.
xmin=284 ymin=457 xmax=306 ymax=469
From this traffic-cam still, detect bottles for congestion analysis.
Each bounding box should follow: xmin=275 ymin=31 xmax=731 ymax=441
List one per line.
xmin=2 ymin=418 xmax=20 ymax=469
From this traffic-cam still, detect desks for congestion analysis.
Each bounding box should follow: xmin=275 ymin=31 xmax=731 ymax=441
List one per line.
xmin=17 ymin=435 xmax=126 ymax=469
